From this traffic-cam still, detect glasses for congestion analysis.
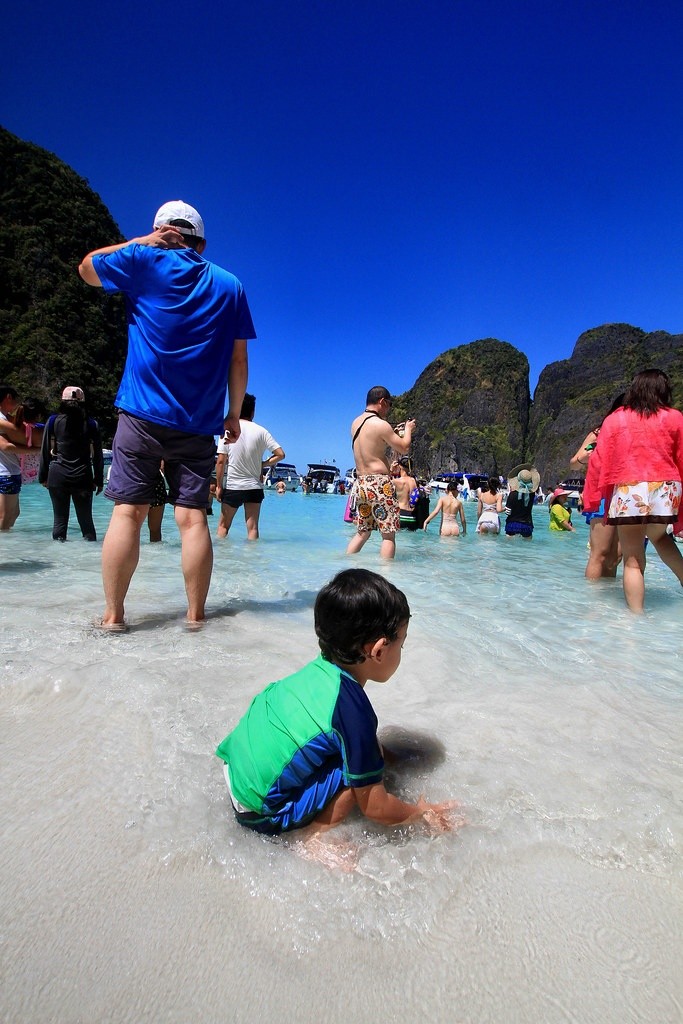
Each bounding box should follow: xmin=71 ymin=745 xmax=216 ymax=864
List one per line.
xmin=380 ymin=397 xmax=393 ymax=407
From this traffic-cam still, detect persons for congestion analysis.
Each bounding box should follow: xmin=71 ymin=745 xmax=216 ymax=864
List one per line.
xmin=77 ymin=199 xmax=257 ymax=624
xmin=505 ymin=463 xmax=540 ymax=538
xmin=569 ymin=369 xmax=683 ymax=612
xmin=346 ymin=386 xmax=415 ymax=558
xmin=534 ymin=483 xmax=585 ymax=511
xmin=216 ymin=568 xmax=413 ymax=834
xmin=549 ymin=488 xmax=573 ymax=531
xmin=206 ymin=393 xmax=285 ymax=540
xmin=389 ymin=456 xmax=419 ymax=531
xmin=39 ymin=386 xmax=105 ymax=540
xmin=275 ymin=478 xmax=286 ymax=493
xmin=301 ymin=477 xmax=352 ymax=495
xmin=474 ymin=477 xmax=512 ymax=534
xmin=107 ymin=459 xmax=169 ymax=542
xmin=0 ymin=385 xmax=54 ymax=530
xmin=423 ymin=482 xmax=466 ymax=535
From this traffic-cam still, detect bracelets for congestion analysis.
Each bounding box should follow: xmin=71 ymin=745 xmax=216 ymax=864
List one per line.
xmin=577 ymin=457 xmax=585 ymax=464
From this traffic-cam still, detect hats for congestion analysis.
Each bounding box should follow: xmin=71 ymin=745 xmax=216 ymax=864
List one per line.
xmin=153 ymin=200 xmax=204 ymax=238
xmin=507 ymin=464 xmax=540 ymax=507
xmin=62 ymin=387 xmax=85 ymax=402
xmin=549 ymin=489 xmax=572 ymax=505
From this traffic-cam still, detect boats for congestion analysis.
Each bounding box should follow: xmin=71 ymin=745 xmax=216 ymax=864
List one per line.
xmin=426 ymin=471 xmax=510 ymax=503
xmin=306 ymin=463 xmax=341 ymax=494
xmin=262 ymin=461 xmax=301 ymax=492
xmin=343 ymin=466 xmax=357 ymax=491
xmin=558 ymin=478 xmax=585 ymax=508
